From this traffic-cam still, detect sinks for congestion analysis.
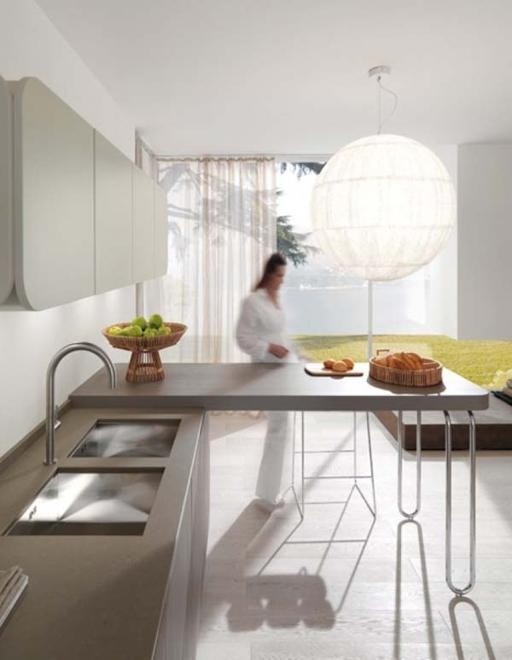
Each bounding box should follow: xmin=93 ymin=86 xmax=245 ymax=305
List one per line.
xmin=71 ymin=417 xmax=181 ymax=457
xmin=6 ymin=467 xmax=165 ymax=536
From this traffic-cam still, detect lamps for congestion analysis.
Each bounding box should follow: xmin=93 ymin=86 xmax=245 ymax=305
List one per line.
xmin=313 ymin=65 xmax=453 ymax=361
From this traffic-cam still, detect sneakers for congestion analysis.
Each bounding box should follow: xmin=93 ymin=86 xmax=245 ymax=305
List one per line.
xmin=272 ymin=493 xmax=287 ymax=507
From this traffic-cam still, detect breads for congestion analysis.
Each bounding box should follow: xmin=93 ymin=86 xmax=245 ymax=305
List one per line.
xmin=374 ymin=351 xmax=439 ymax=369
xmin=332 ymin=361 xmax=347 ymax=373
xmin=341 ymin=358 xmax=354 ymax=369
xmin=323 ymin=359 xmax=334 ymax=369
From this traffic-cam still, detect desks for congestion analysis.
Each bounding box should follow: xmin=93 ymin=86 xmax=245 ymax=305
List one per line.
xmin=67 ymin=362 xmax=489 ymax=596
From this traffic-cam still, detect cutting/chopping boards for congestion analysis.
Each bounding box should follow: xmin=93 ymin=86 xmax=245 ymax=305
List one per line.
xmin=306 ymin=362 xmax=365 ymax=378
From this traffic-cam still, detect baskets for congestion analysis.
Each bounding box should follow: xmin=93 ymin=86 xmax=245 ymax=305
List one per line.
xmin=369 ymin=349 xmax=443 ymax=387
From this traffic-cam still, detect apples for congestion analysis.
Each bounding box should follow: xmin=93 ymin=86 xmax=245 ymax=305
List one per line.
xmin=149 ymin=313 xmax=162 ymax=328
xmin=158 ymin=328 xmax=171 ymax=334
xmin=143 ymin=328 xmax=157 ymax=336
xmin=132 ymin=317 xmax=145 ymax=329
xmin=108 ymin=327 xmax=121 ymax=337
xmin=121 ymin=325 xmax=142 ymax=337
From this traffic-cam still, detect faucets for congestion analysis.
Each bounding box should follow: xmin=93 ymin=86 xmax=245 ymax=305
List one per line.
xmin=44 ymin=343 xmax=117 ymax=464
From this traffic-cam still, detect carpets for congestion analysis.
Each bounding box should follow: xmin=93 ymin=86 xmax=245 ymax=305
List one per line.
xmin=288 ymin=333 xmax=512 ymax=391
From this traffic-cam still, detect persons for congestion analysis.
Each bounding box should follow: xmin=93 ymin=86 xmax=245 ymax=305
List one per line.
xmin=235 ymin=253 xmax=294 ymax=509
